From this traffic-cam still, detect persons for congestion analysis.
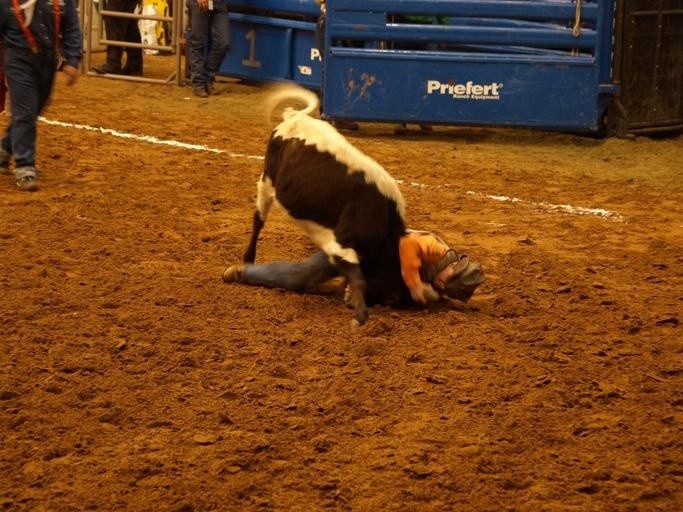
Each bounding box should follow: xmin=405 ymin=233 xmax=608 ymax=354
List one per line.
xmin=91 ymin=0 xmax=143 ymax=76
xmin=313 ymin=0 xmax=365 ymax=131
xmin=379 ymin=10 xmax=452 ymax=137
xmin=186 ymin=0 xmax=231 ymax=98
xmin=0 ymin=0 xmax=83 ymax=192
xmin=222 ymin=228 xmax=485 ymax=311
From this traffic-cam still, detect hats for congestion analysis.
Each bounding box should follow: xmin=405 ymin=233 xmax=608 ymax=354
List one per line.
xmin=442 ymin=252 xmax=486 ymax=304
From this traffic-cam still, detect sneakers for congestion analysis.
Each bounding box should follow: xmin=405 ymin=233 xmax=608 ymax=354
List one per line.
xmin=15 ymin=176 xmax=39 ymax=193
xmin=193 ymin=85 xmax=209 ymax=98
xmin=92 ymin=61 xmax=122 ymax=75
xmin=206 ymin=83 xmax=221 ymax=96
xmin=0 ymin=139 xmax=12 ymax=175
xmin=221 ymin=261 xmax=249 ymax=284
xmin=117 ymin=65 xmax=144 ymax=76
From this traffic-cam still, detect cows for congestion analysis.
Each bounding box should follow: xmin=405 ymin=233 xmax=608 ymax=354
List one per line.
xmin=240 ymin=81 xmax=440 ymax=330
